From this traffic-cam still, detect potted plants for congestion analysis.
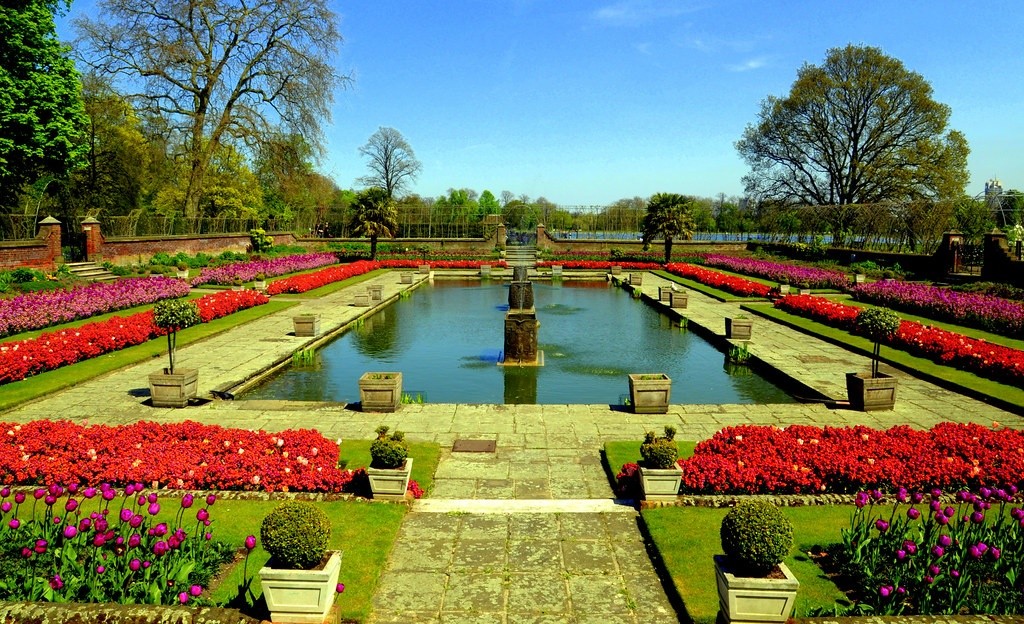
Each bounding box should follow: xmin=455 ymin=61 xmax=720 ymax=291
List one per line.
xmin=853 ymin=266 xmax=866 ymax=282
xmin=797 ymin=280 xmax=811 ymax=296
xmin=725 ymin=314 xmax=752 ymax=339
xmin=292 ymin=312 xmax=322 ymax=337
xmin=638 ymin=425 xmax=683 ymax=500
xmin=883 ymin=270 xmax=895 ymax=282
xmin=254 ymin=274 xmax=266 ymax=289
xmin=359 ymin=372 xmax=402 ymax=413
xmin=610 ymin=247 xmax=624 ymax=275
xmin=231 ymin=276 xmax=245 ymax=291
xmin=417 ymin=243 xmax=430 ymax=274
xmin=778 ymin=276 xmax=790 ymax=294
xmin=258 ymin=503 xmax=343 ymax=624
xmin=713 ymin=502 xmax=799 ymax=624
xmin=658 ymin=285 xmax=689 ymax=308
xmin=846 ymin=305 xmax=900 ymax=411
xmin=628 ymin=373 xmax=671 ymax=413
xmin=176 ymin=262 xmax=189 ymax=278
xmin=148 ymin=298 xmax=201 ymax=407
xmin=366 ymin=425 xmax=414 ymax=500
